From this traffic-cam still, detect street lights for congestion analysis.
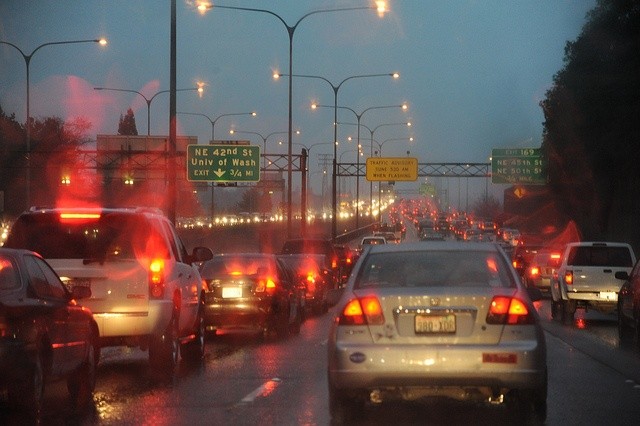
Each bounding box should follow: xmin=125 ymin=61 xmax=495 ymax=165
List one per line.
xmin=194 ymin=1 xmax=390 ymax=242
xmin=124 ymin=180 xmax=134 ymax=208
xmin=357 ymin=144 xmax=410 ymax=218
xmin=347 ymin=136 xmax=415 ymax=221
xmin=272 ymin=73 xmax=400 ymax=241
xmin=230 ymin=130 xmax=301 ymax=211
xmin=94 ymin=87 xmax=204 ymax=137
xmin=278 ymin=140 xmax=338 ymax=210
xmin=174 ymin=111 xmax=257 ymax=225
xmin=0 ymin=38 xmax=107 ymax=211
xmin=311 ymin=103 xmax=408 ymax=229
xmin=61 ymin=180 xmax=70 ymax=210
xmin=332 ymin=120 xmax=412 ymax=224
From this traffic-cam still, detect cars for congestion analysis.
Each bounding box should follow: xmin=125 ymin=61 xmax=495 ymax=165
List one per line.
xmin=550 ymin=241 xmax=637 ymax=327
xmin=512 ymin=245 xmax=545 ymax=277
xmin=522 ymin=253 xmax=564 ymax=302
xmin=1 ymin=247 xmax=101 ymax=425
xmin=614 ymin=256 xmax=639 ymax=354
xmin=362 ymin=197 xmax=525 ymax=244
xmin=176 ymin=200 xmax=394 ymax=229
xmin=200 ymin=252 xmax=307 ymax=345
xmin=334 ymin=244 xmax=354 ymax=284
xmin=277 ymin=254 xmax=334 ymax=316
xmin=328 ymin=241 xmax=548 ymax=426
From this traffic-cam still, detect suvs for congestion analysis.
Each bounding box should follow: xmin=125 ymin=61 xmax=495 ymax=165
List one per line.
xmin=281 ymin=238 xmax=342 ymax=306
xmin=3 ymin=206 xmax=210 ymax=381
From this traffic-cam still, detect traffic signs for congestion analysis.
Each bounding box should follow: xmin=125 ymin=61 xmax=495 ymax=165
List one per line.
xmin=186 ymin=144 xmax=260 ymax=182
xmin=366 ymin=158 xmax=418 ymax=182
xmin=492 ymin=156 xmax=550 ymax=184
xmin=492 ymin=148 xmax=545 ymax=157
